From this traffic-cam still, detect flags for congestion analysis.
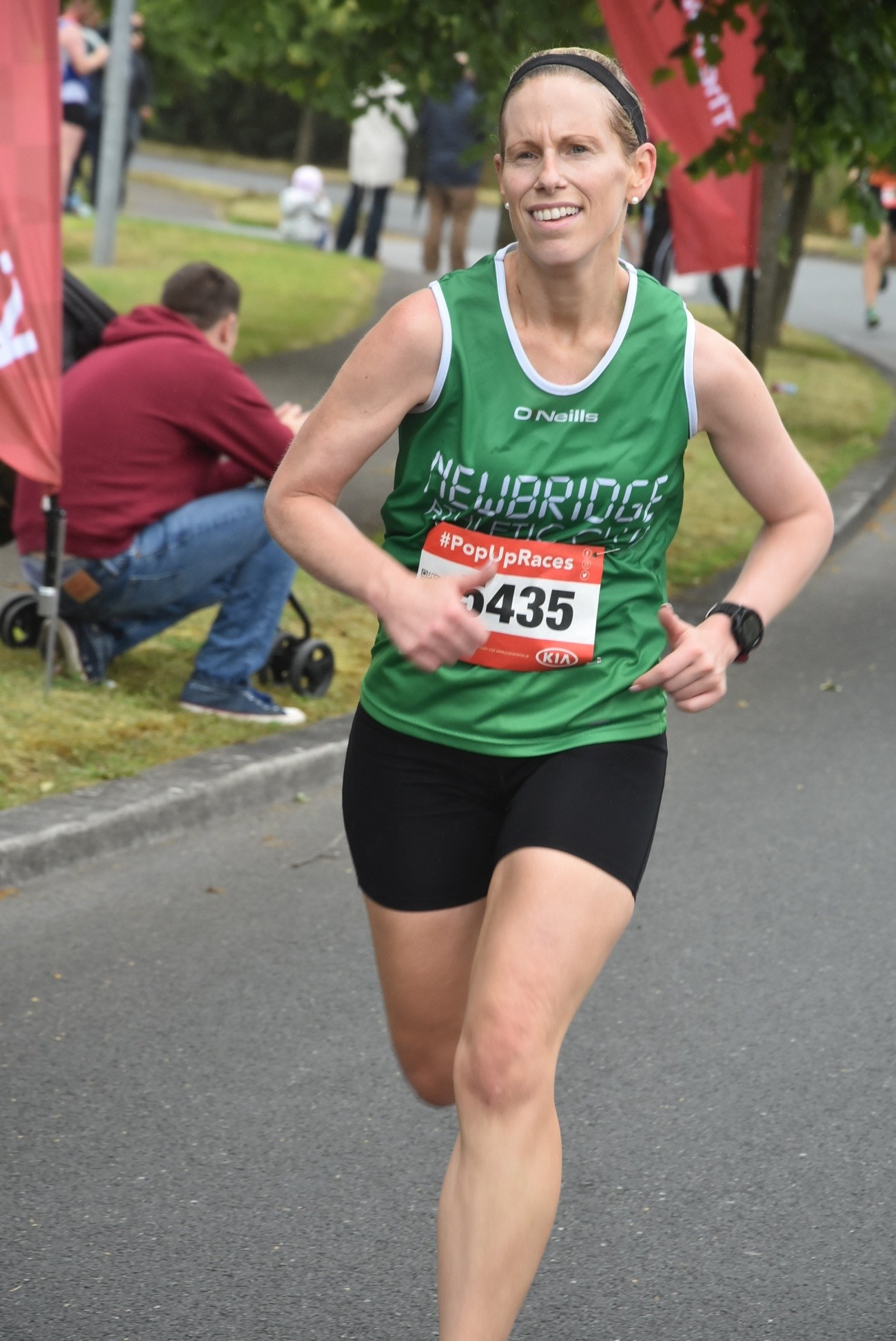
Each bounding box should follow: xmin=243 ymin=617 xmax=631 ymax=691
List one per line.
xmin=596 ymin=0 xmax=760 ymax=274
xmin=0 ymin=0 xmax=61 ymax=484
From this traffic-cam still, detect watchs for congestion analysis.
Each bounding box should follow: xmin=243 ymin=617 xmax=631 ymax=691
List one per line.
xmin=704 ymin=602 xmax=764 ymax=662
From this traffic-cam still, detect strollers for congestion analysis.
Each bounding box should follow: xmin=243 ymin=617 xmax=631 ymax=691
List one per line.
xmin=0 ymin=266 xmax=335 ymax=698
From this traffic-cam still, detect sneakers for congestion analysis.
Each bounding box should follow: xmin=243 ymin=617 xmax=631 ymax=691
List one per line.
xmin=176 ymin=668 xmax=306 ymax=724
xmin=38 ymin=615 xmax=119 ymax=690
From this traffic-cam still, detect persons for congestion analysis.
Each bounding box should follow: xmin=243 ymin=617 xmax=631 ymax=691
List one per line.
xmin=53 ymin=0 xmax=151 ymax=216
xmin=274 ymin=50 xmax=486 ymax=277
xmin=12 ymin=263 xmax=306 ymax=727
xmin=622 ymin=188 xmax=676 ymax=286
xmin=263 ymin=47 xmax=831 ymax=1341
xmin=857 ymin=170 xmax=896 ymax=329
